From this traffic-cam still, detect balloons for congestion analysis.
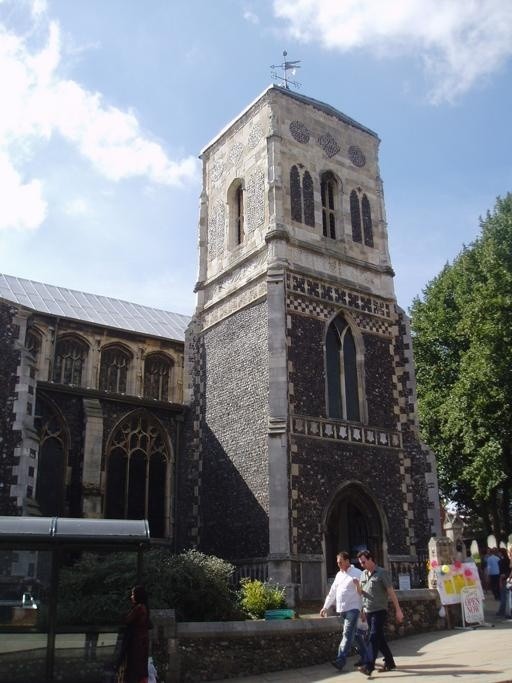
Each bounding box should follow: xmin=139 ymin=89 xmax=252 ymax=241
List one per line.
xmin=439 ymin=605 xmax=446 ymax=618
xmin=464 ymin=570 xmax=473 ymax=576
xmin=441 ymin=564 xmax=449 ymax=573
xmin=455 ymin=560 xmax=462 ymax=568
xmin=430 ymin=560 xmax=437 ymax=568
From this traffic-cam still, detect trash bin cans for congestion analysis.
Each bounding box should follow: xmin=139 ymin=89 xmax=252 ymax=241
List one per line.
xmin=264 ymin=609 xmax=295 ymax=620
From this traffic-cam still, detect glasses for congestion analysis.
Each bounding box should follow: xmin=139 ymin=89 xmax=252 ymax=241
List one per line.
xmin=360 ymin=561 xmax=366 ymax=564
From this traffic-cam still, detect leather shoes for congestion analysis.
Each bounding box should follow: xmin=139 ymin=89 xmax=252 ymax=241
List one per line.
xmin=354 ymin=661 xmax=375 ymax=675
xmin=378 ymin=662 xmax=396 ymax=672
xmin=331 ymin=660 xmax=344 ymax=671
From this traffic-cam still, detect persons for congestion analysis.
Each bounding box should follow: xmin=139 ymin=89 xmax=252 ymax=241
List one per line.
xmin=353 ymin=550 xmax=404 ymax=676
xmin=320 ymin=549 xmax=370 ymax=671
xmin=481 ymin=546 xmax=512 ymax=619
xmin=124 ymin=584 xmax=150 ymax=683
